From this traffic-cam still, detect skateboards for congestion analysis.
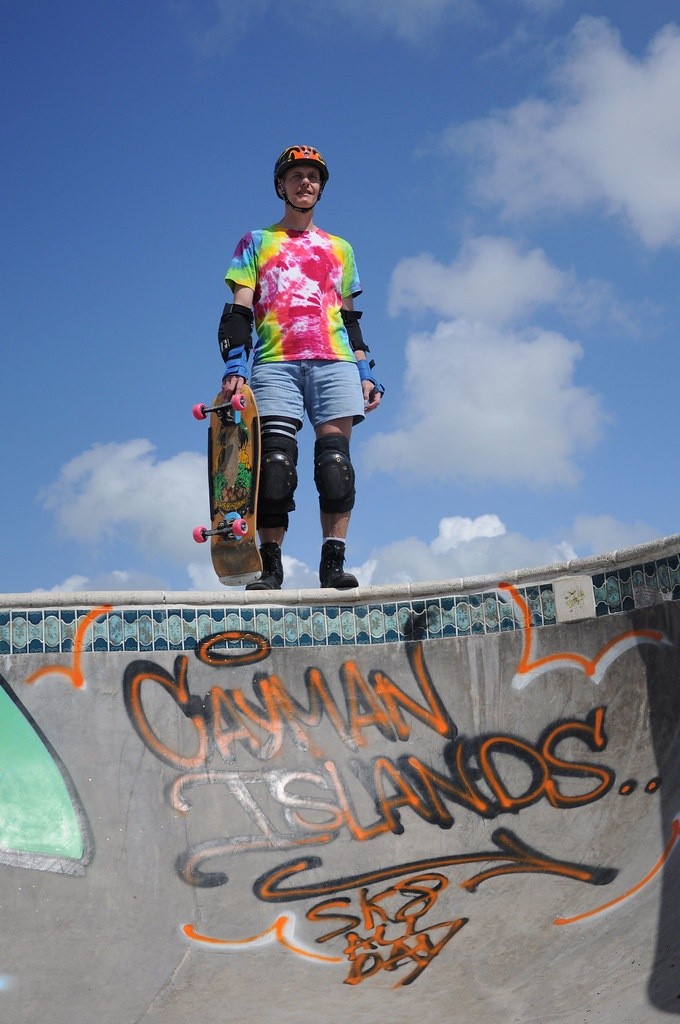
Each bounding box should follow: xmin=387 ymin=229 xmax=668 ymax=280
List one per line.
xmin=192 ymin=383 xmax=264 ymax=586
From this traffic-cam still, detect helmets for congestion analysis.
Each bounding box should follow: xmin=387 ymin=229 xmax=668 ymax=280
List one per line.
xmin=273 ymin=145 xmax=329 ymax=201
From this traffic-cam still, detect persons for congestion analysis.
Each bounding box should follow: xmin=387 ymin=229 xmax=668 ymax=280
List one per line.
xmin=218 ymin=145 xmax=384 ymax=591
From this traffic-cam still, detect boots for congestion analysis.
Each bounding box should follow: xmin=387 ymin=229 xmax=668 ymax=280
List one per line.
xmin=319 ymin=540 xmax=359 ymax=588
xmin=245 ymin=541 xmax=284 ymax=590
